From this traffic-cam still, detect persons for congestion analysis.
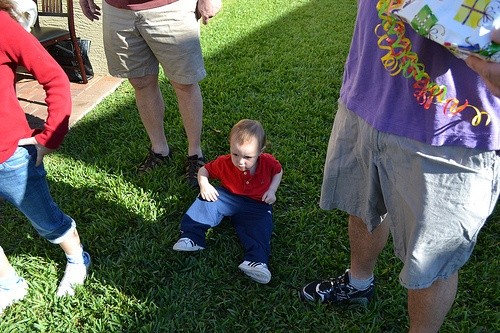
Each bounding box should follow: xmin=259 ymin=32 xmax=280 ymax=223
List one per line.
xmin=303 ymin=0 xmax=500 ymax=333
xmin=77 ymin=0 xmax=222 ymax=187
xmin=173 ymin=118 xmax=282 ymax=284
xmin=0 ymin=0 xmax=91 ymax=312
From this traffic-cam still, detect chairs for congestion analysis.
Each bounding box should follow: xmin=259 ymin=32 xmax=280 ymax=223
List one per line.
xmin=29 ymin=0 xmax=88 ymax=83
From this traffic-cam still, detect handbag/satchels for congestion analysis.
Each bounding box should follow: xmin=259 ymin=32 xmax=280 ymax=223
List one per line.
xmin=46 ymin=38 xmax=94 ymax=82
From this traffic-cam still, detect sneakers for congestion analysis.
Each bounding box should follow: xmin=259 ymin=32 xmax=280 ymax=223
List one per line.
xmin=238 ymin=260 xmax=272 ymax=284
xmin=303 ymin=268 xmax=374 ymax=306
xmin=0 ymin=276 xmax=28 ymax=314
xmin=56 ymin=251 xmax=91 ymax=298
xmin=136 ymin=147 xmax=173 ymax=176
xmin=172 ymin=237 xmax=204 ymax=251
xmin=184 ymin=154 xmax=206 ymax=189
